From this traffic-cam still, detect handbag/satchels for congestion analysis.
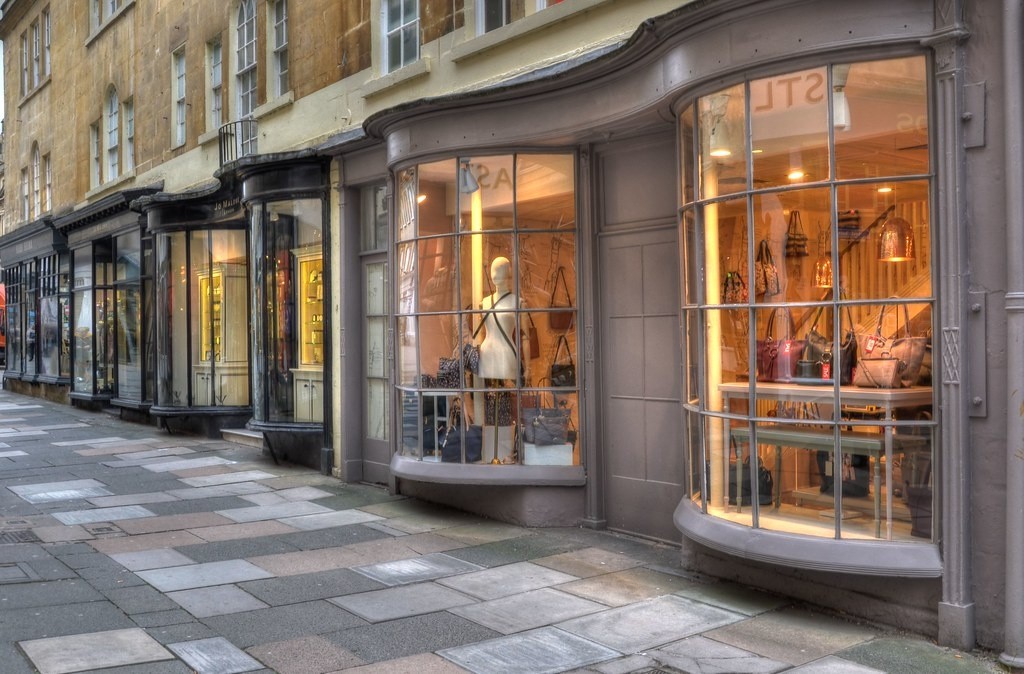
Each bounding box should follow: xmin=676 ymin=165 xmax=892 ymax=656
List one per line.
xmin=816 ymin=410 xmax=870 ymax=498
xmin=756 ymin=287 xmax=929 ymax=388
xmin=905 ymin=458 xmax=932 ymax=539
xmin=401 ymin=303 xmax=577 ymax=464
xmin=722 ymin=209 xmax=861 ymax=304
xmin=548 ymin=266 xmax=573 ymax=330
xmin=700 ymin=429 xmax=773 ymax=506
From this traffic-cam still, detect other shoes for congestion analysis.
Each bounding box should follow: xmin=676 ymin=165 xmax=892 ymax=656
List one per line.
xmin=29 ymin=358 xmax=33 ymax=361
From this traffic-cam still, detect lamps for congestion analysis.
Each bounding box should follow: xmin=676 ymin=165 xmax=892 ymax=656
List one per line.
xmin=460 ymin=157 xmax=479 ymax=195
xmin=708 ymin=94 xmax=732 ymax=157
xmin=877 ymin=138 xmax=916 ymax=263
xmin=826 ymin=64 xmax=851 ymax=132
xmin=811 ymin=161 xmax=833 ymax=288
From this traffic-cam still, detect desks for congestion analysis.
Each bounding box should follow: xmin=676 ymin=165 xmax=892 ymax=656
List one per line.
xmin=720 ymin=380 xmax=933 ymax=540
xmin=414 ymin=388 xmax=472 ymax=463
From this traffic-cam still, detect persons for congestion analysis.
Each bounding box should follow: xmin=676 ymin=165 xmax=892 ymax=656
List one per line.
xmin=478 ymin=257 xmax=530 ymax=388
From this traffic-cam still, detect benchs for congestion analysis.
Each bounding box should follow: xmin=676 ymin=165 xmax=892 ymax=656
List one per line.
xmin=731 ymin=425 xmax=927 ymax=538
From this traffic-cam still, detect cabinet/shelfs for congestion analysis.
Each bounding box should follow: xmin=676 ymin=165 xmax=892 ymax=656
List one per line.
xmin=289 ymin=244 xmax=323 ymax=423
xmin=192 ymin=262 xmax=247 ymax=407
